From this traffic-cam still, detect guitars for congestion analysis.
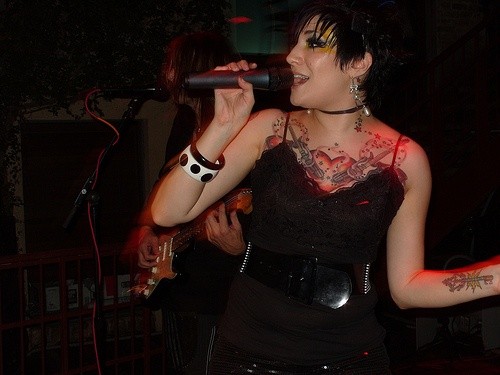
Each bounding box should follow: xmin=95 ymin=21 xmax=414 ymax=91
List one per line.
xmin=129 ymin=188 xmax=253 ymax=302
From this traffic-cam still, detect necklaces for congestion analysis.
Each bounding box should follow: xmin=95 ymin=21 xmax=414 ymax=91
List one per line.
xmin=319 ymin=103 xmax=369 ymax=114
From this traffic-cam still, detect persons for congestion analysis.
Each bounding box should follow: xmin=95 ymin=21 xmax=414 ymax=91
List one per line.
xmin=149 ymin=0 xmax=500 ymax=375
xmin=128 ymin=31 xmax=256 ymax=375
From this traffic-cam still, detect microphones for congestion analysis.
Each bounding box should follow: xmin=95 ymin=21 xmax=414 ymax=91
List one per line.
xmin=102 ymin=78 xmax=169 ymax=101
xmin=176 ymin=63 xmax=294 ymax=91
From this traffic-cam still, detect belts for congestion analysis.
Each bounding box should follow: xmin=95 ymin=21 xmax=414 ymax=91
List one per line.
xmin=245 ymin=244 xmax=380 ymax=305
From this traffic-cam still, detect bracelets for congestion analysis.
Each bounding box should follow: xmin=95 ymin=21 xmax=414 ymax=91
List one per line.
xmin=177 ymin=141 xmax=225 ymax=183
